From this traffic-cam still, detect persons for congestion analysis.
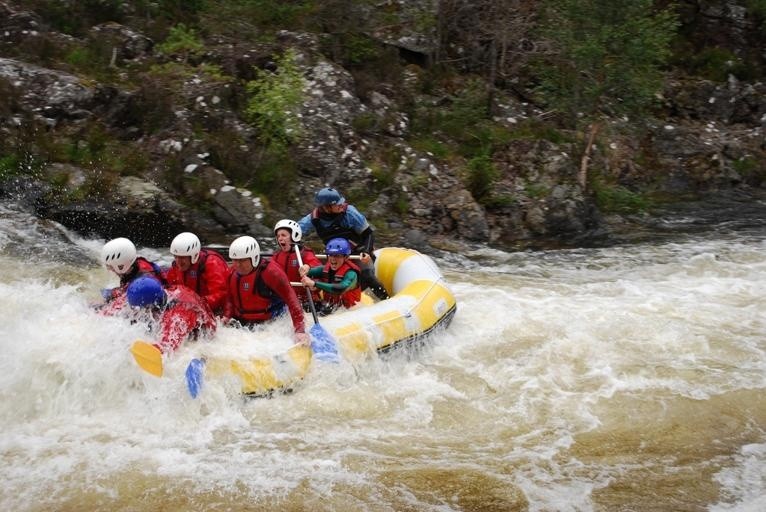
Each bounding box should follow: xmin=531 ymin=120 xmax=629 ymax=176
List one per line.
xmin=163 ymin=232 xmax=230 ymax=318
xmin=269 ymin=219 xmax=324 ymax=304
xmin=297 ymin=186 xmax=390 ymax=300
xmin=126 ymin=274 xmax=215 ymax=355
xmin=298 ymin=237 xmax=362 ymax=310
xmin=100 ymin=236 xmax=168 ymax=303
xmin=216 ymin=234 xmax=311 ymax=345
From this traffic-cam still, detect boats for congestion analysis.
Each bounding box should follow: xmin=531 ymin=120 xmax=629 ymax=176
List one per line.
xmin=79 ymin=247 xmax=456 ymax=396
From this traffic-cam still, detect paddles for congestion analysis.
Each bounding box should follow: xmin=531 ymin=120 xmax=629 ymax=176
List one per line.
xmin=186 ymin=359 xmax=202 ymax=398
xmin=129 ymin=340 xmax=163 ymax=378
xmin=291 ymin=242 xmax=339 ymax=364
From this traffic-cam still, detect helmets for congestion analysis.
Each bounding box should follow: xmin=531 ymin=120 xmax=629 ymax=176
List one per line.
xmin=315 ymin=188 xmax=346 ymax=206
xmin=170 ymin=232 xmax=201 ymax=264
xmin=101 ymin=237 xmax=138 ymax=275
xmin=229 ymin=236 xmax=260 ymax=268
xmin=274 ymin=219 xmax=302 ymax=242
xmin=127 ymin=277 xmax=167 ymax=312
xmin=324 ymin=238 xmax=351 ymax=256
